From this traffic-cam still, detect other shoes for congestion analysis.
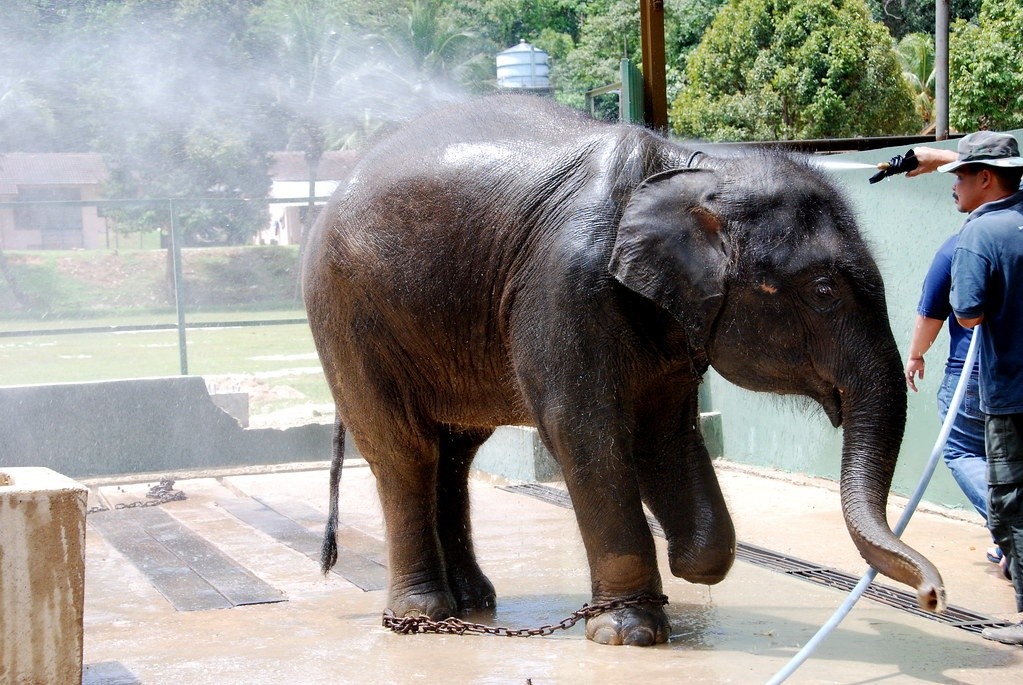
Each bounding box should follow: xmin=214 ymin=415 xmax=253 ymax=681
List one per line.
xmin=981 ymin=623 xmax=1023 ymax=644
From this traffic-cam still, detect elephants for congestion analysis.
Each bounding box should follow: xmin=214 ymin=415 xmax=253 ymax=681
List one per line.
xmin=298 ymin=92 xmax=947 ymax=647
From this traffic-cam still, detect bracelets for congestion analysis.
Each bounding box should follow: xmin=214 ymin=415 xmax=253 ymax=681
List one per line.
xmin=910 ymin=356 xmax=923 ymax=363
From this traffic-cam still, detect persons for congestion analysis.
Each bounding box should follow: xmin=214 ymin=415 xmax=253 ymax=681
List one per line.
xmin=877 ymin=126 xmax=1022 ymax=645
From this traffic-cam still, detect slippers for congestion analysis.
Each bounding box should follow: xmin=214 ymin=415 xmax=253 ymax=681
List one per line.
xmin=986 ymin=547 xmax=1003 ymax=563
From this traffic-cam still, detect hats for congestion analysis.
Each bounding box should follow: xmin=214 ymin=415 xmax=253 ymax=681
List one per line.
xmin=936 ymin=131 xmax=1023 ymax=173
